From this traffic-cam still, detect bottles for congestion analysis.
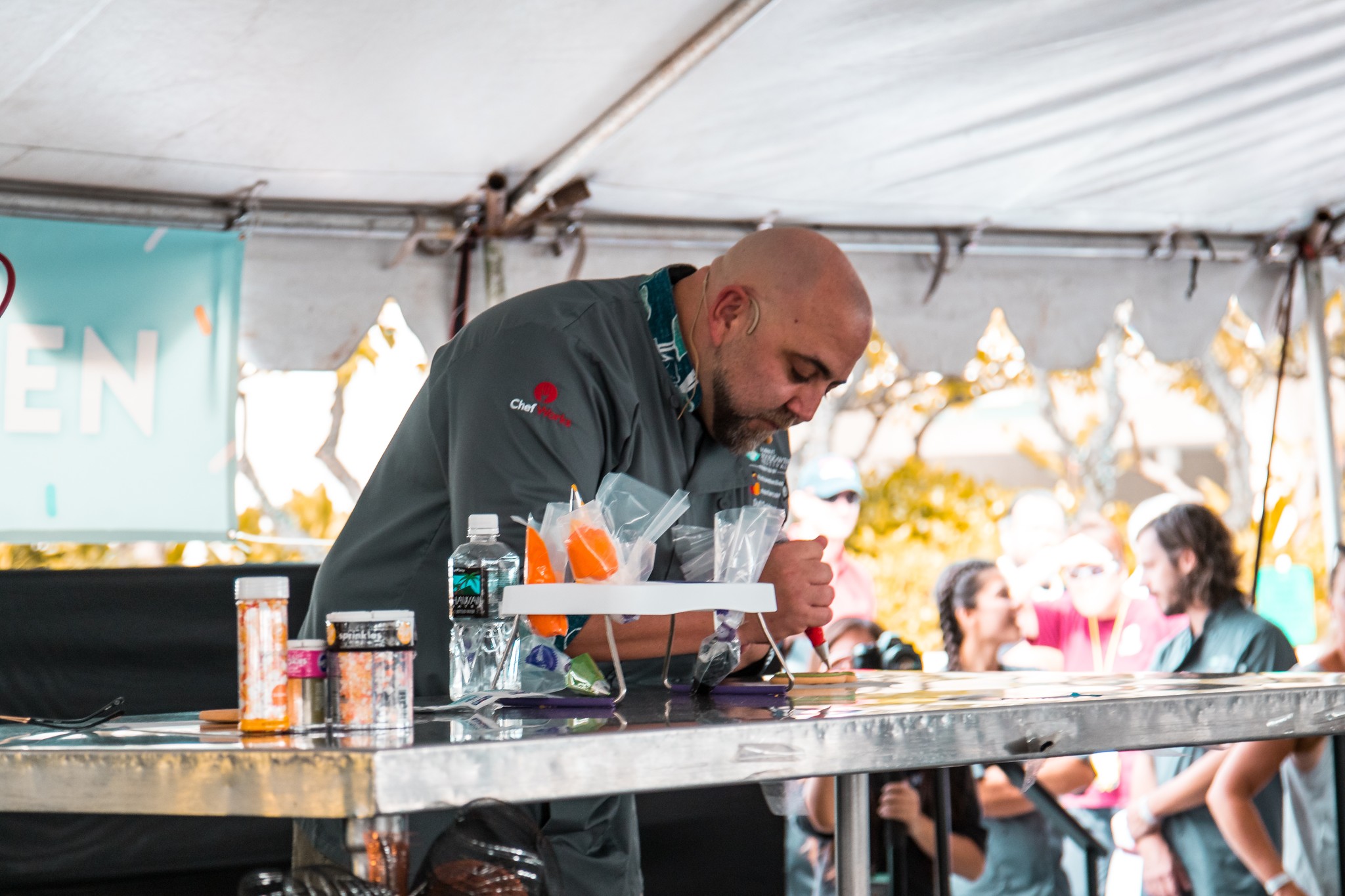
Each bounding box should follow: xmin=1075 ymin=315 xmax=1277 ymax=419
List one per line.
xmin=231 ymin=571 xmax=291 ymax=732
xmin=447 ymin=512 xmax=523 ymax=701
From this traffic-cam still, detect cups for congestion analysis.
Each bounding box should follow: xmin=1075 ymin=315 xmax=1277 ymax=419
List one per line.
xmin=326 ymin=605 xmax=415 ymax=731
xmin=284 ymin=633 xmax=330 ymax=729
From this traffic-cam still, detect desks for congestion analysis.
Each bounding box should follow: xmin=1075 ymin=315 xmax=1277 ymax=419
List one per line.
xmin=0 ymin=663 xmax=1344 ymax=891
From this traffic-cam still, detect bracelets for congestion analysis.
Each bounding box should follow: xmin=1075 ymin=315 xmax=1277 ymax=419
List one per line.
xmin=1263 ymin=873 xmax=1294 ymax=895
xmin=1136 ymin=794 xmax=1162 ymax=831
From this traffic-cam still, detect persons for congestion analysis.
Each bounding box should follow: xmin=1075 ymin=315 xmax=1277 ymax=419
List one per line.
xmin=780 ymin=620 xmax=991 ymax=896
xmin=1204 ymin=545 xmax=1345 ymax=896
xmin=926 ymin=559 xmax=1093 ymax=896
xmin=1098 ymin=505 xmax=1299 ymax=896
xmin=784 ymin=451 xmax=875 ymax=716
xmin=292 ymin=227 xmax=877 ymax=896
xmin=998 ymin=523 xmax=1185 ymax=896
